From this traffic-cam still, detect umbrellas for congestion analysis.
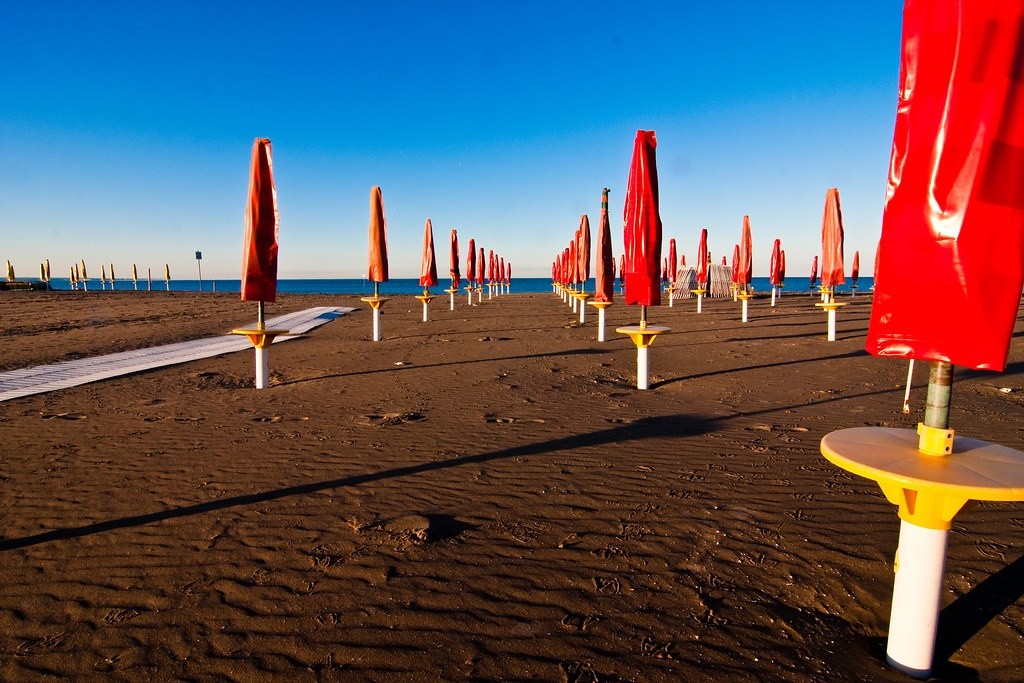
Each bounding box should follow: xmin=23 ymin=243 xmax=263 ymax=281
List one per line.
xmin=494 ymin=254 xmax=500 ymax=283
xmin=6 ymin=259 xmax=171 ymax=284
xmin=466 ymin=238 xmax=476 ymax=287
xmin=449 ymin=228 xmax=461 ymax=294
xmin=621 ymin=128 xmax=664 ymax=323
xmin=477 ymin=247 xmax=486 ymax=288
xmin=730 ymin=215 xmax=753 ymax=292
xmin=864 ymin=0 xmax=1024 ymax=431
xmin=506 ymin=262 xmax=512 ymax=284
xmin=819 ymin=187 xmax=845 ymax=300
xmin=368 ymin=185 xmax=389 ymax=294
xmin=610 ymin=227 xmax=860 ymax=288
xmin=499 ymin=257 xmax=505 ymax=282
xmin=240 ymin=136 xmax=280 ymax=322
xmin=551 ymin=214 xmax=591 ymax=292
xmin=488 ymin=249 xmax=495 ymax=284
xmin=418 ymin=218 xmax=439 ymax=291
xmin=593 ymin=188 xmax=616 ymax=303
